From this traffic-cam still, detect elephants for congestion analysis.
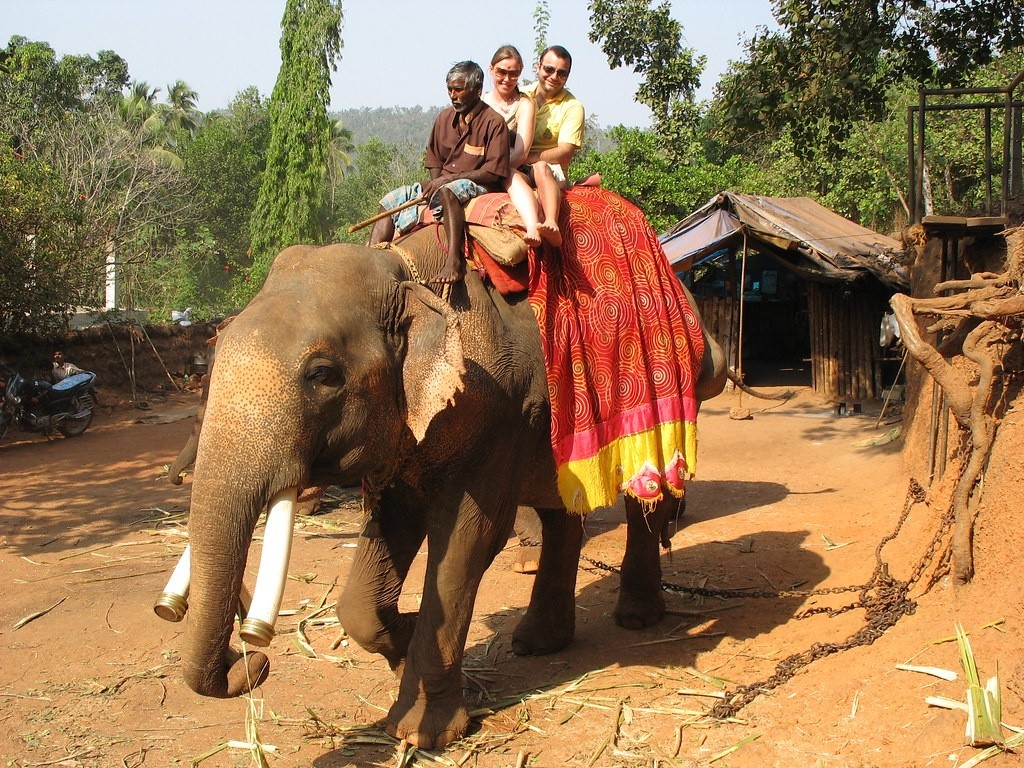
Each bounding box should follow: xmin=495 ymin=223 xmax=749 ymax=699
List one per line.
xmin=155 ymin=186 xmax=729 ymax=748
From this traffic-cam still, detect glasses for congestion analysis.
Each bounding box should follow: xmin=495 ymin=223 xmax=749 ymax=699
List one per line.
xmin=493 ymin=67 xmax=522 ymax=79
xmin=541 ymin=64 xmax=570 ymax=78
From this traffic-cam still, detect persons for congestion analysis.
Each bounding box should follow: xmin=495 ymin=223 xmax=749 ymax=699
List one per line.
xmin=525 ymin=45 xmax=584 ymax=247
xmin=369 ymin=61 xmax=510 ymax=283
xmin=45 ymin=348 xmax=84 ymax=384
xmin=481 ymin=45 xmax=541 ymax=248
